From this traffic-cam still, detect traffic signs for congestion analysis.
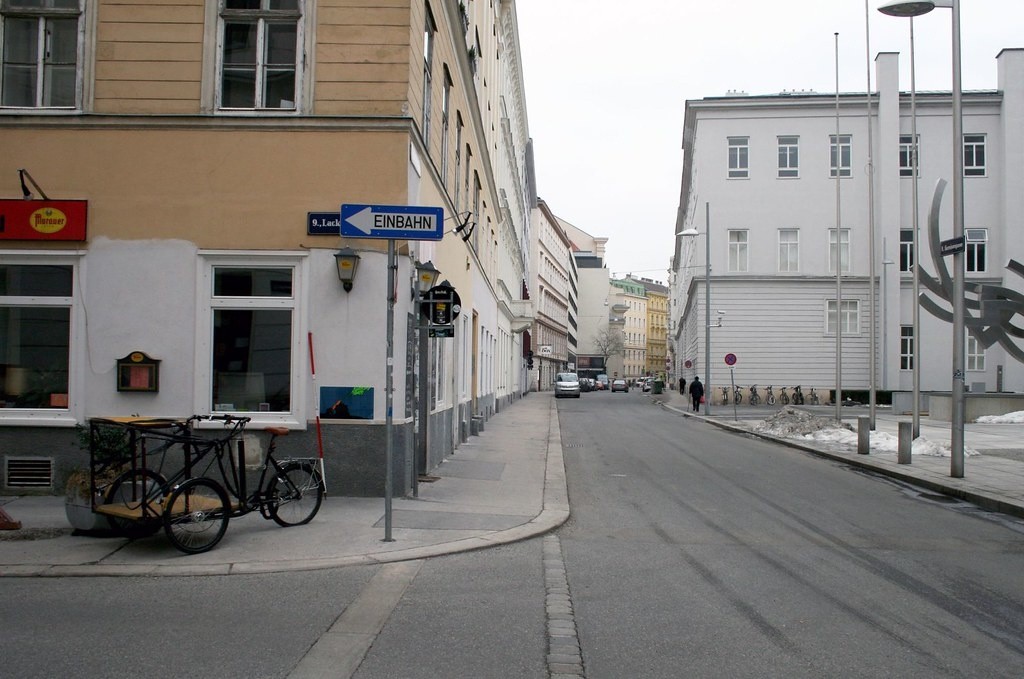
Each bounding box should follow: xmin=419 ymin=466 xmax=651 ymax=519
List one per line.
xmin=340 ymin=202 xmax=444 ymax=241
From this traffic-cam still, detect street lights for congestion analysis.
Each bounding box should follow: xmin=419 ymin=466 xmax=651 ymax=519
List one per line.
xmin=878 ymin=0 xmax=965 ymax=480
xmin=676 ymin=228 xmax=713 ymax=416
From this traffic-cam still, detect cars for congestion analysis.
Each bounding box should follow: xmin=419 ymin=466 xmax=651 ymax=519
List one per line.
xmin=641 ymin=376 xmax=657 ymax=392
xmin=595 ymin=381 xmax=604 ymax=391
xmin=580 ymin=378 xmax=591 ymax=390
xmin=589 ymin=378 xmax=597 ymax=391
xmin=611 ymin=380 xmax=629 ymax=393
xmin=636 ymin=377 xmax=648 ymax=388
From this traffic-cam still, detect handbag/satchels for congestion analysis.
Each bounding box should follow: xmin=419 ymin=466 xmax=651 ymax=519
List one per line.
xmin=697 ymin=409 xmax=699 ymax=411
xmin=700 ymin=395 xmax=705 ymax=403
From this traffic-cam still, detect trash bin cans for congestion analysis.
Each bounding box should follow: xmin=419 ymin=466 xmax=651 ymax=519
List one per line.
xmin=651 ymin=380 xmax=664 ymax=394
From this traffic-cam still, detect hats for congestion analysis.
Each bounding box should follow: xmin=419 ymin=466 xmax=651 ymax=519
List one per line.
xmin=695 ymin=376 xmax=699 ymax=380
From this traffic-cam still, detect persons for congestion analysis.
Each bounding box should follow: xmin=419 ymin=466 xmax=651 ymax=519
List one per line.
xmin=679 ymin=376 xmax=686 ymax=396
xmin=689 ymin=376 xmax=704 ymax=412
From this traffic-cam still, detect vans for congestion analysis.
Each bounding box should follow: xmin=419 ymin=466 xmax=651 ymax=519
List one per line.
xmin=596 ymin=374 xmax=610 ymax=390
xmin=553 ymin=372 xmax=581 ymax=398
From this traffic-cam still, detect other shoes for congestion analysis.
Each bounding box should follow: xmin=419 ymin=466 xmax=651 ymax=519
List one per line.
xmin=693 ymin=408 xmax=695 ymax=411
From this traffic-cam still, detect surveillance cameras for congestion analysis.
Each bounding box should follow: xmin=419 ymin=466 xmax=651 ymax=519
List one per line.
xmin=717 ymin=310 xmax=726 ymax=315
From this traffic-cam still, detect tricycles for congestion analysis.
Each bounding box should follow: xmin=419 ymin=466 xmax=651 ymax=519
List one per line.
xmin=88 ymin=412 xmax=327 ymax=555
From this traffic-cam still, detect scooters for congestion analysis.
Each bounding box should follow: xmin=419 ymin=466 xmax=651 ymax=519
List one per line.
xmin=748 ymin=384 xmax=761 ymax=406
xmin=764 ymin=385 xmax=775 ymax=405
xmin=733 ymin=384 xmax=744 ymax=404
xmin=780 ymin=387 xmax=790 ymax=405
xmin=718 ymin=386 xmax=731 ymax=405
xmin=790 ymin=383 xmax=804 ymax=405
xmin=804 ymin=384 xmax=819 ymax=406
